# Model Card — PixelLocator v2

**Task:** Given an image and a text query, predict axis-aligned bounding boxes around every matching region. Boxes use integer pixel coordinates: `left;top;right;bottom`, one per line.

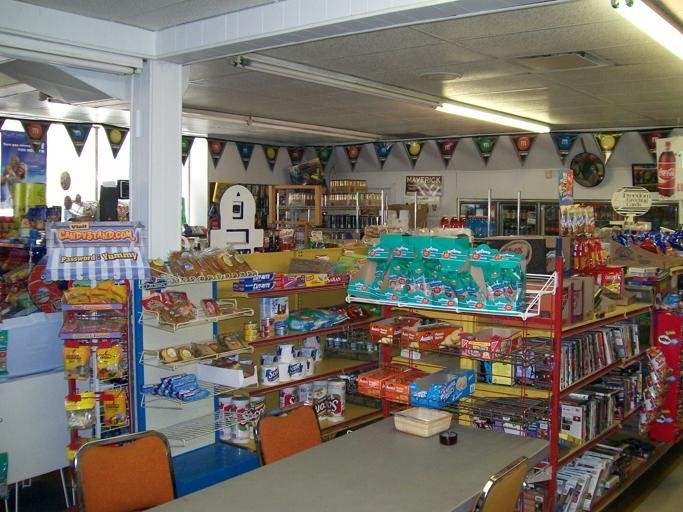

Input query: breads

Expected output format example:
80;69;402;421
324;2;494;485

159;334;245;364
149;257;167;282
171;247;255;278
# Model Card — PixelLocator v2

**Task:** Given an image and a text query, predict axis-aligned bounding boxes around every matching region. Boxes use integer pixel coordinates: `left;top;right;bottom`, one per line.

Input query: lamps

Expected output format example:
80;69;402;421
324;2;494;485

234;54;438;106
614;0;683;61
434;98;553;137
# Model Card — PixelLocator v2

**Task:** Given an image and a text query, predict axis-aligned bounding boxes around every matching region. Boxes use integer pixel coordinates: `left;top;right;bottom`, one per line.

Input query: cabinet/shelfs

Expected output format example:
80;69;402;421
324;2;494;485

0;181;683;512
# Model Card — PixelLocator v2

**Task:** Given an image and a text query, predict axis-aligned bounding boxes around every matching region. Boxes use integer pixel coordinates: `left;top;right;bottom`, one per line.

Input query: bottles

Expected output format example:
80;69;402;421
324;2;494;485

503;209;535;236
440;215;497;238
288;179;382;209
657;141;676;197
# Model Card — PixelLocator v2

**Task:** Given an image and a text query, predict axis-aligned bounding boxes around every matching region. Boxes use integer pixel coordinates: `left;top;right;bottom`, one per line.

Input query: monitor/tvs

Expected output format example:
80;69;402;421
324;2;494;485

632;162;659;192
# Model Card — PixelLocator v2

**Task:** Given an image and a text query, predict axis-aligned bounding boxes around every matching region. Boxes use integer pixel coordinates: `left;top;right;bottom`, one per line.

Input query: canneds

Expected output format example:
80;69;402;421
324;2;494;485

242;318;275;343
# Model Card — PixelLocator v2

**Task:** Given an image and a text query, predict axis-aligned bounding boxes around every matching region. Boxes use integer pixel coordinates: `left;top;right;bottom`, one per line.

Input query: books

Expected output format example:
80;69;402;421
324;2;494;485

627;266;661;286
472;315;657;512
